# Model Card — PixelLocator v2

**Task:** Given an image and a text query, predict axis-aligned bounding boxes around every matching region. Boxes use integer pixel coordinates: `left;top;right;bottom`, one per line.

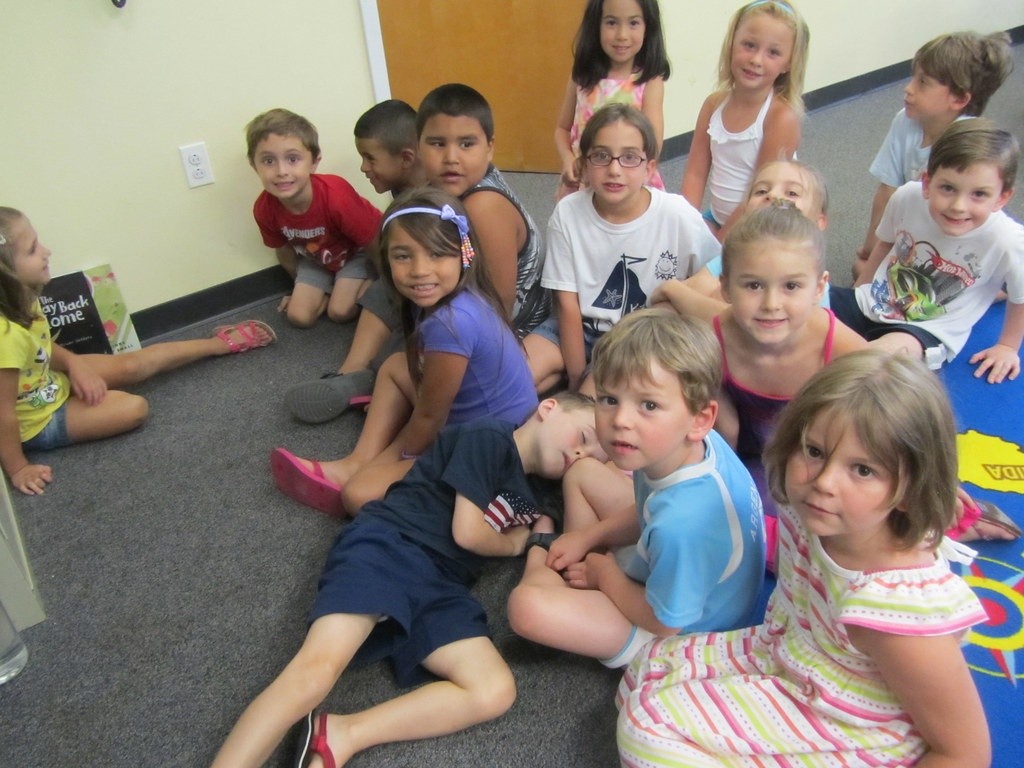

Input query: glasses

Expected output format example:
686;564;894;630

583;150;644;168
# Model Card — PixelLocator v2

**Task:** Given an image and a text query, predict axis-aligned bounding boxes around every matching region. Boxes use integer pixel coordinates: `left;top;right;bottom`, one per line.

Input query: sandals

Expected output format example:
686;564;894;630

213;319;277;353
293;708;337;768
972;500;1022;542
525;505;563;551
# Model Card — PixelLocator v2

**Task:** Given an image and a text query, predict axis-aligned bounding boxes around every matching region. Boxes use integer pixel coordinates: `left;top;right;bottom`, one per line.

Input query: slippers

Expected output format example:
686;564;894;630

272;446;346;518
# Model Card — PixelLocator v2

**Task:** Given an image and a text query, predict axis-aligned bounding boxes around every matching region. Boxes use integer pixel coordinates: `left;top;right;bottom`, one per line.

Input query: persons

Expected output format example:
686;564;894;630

211;3;1024;768
1;206;277;495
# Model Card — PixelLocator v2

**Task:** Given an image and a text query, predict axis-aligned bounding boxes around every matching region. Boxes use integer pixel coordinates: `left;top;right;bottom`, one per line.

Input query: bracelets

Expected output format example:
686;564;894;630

526;533;560;551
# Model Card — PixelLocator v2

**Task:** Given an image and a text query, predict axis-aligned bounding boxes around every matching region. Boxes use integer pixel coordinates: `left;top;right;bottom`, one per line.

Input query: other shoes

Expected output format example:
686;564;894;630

285;370;377;424
0;646;29;685
